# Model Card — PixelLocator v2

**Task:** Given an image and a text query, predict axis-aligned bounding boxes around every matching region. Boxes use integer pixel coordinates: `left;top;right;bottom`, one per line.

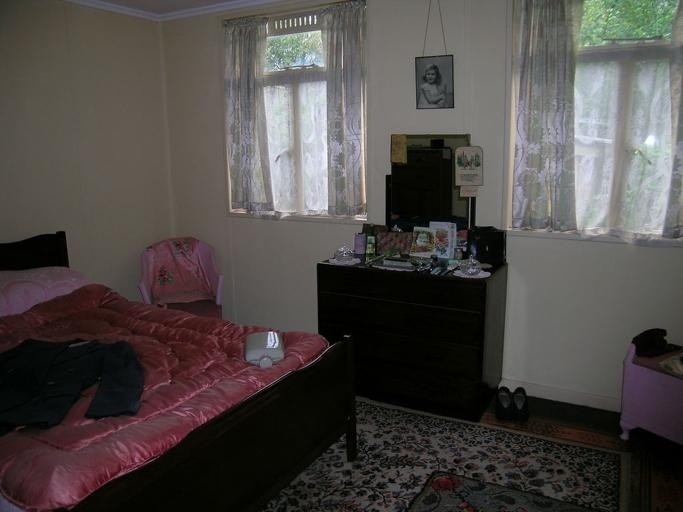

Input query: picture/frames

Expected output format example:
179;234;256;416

414;54;455;109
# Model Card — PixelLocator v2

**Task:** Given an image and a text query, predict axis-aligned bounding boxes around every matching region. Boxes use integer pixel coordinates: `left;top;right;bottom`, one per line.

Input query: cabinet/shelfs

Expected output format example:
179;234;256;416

316;260;509;422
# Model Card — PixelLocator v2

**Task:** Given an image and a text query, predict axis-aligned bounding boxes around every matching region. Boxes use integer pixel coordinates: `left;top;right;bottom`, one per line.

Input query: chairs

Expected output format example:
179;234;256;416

135;236;223;322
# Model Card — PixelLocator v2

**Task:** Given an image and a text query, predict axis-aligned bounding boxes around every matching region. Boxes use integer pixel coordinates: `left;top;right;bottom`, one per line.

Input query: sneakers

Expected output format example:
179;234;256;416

495;387;530;424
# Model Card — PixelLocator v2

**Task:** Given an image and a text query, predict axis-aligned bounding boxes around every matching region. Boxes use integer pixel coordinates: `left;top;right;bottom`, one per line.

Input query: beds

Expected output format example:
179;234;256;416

0;231;359;512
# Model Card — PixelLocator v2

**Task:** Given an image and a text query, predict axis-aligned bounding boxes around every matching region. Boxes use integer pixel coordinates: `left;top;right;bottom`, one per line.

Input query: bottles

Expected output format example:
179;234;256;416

243;331;285;369
364;224;376;256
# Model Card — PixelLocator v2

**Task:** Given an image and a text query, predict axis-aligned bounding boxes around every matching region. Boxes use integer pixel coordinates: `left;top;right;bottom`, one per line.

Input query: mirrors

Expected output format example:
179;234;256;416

390;133;472;229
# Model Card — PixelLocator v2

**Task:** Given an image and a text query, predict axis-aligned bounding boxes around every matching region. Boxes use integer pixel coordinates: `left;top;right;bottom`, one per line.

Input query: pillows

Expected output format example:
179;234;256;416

0;267;86;317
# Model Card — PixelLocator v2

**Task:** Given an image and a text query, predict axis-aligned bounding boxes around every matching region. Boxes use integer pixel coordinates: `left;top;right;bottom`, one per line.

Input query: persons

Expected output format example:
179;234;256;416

415;64;450;108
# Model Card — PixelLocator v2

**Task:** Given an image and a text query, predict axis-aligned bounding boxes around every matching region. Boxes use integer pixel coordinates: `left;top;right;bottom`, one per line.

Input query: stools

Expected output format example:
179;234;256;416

619;327;683;444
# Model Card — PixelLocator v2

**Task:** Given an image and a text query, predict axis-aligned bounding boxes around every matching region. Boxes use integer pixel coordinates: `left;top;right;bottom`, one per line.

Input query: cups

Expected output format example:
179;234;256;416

353;233;366;255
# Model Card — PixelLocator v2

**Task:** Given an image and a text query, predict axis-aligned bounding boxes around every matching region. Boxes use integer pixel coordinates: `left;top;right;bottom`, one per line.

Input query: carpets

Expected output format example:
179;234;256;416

261;396;631;512
